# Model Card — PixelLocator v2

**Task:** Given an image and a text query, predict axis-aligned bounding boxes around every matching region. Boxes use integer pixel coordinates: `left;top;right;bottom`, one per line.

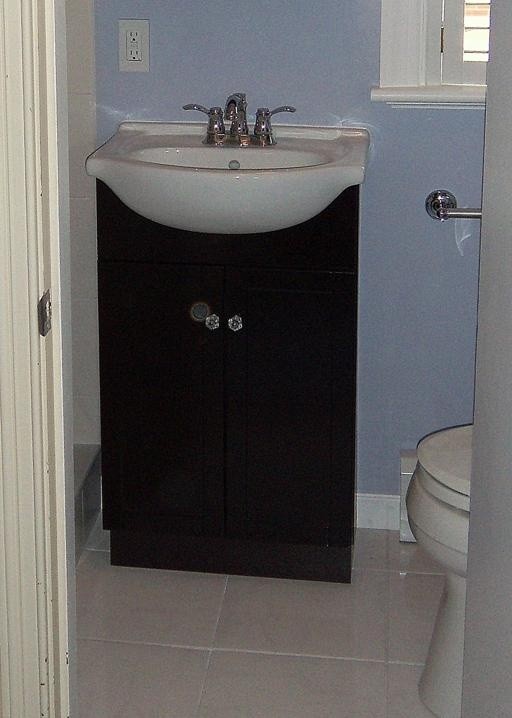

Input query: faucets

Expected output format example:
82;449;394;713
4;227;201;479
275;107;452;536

223;91;250;139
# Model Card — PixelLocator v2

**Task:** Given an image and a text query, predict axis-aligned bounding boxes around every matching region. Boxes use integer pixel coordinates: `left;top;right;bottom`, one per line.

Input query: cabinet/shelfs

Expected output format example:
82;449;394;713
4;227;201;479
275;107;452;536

96;177;361;583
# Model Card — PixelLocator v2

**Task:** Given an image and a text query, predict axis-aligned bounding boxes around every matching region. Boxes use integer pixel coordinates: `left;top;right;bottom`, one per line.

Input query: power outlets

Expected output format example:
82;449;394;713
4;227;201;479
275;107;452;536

116;19;152;73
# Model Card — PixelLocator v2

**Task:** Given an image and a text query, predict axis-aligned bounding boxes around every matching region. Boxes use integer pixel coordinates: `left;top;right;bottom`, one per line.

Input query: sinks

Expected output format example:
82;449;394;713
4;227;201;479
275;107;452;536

85;122;369;235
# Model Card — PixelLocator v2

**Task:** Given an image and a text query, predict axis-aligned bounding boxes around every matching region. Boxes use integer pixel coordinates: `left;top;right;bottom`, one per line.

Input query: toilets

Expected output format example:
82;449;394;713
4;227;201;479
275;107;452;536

406;420;473;718
